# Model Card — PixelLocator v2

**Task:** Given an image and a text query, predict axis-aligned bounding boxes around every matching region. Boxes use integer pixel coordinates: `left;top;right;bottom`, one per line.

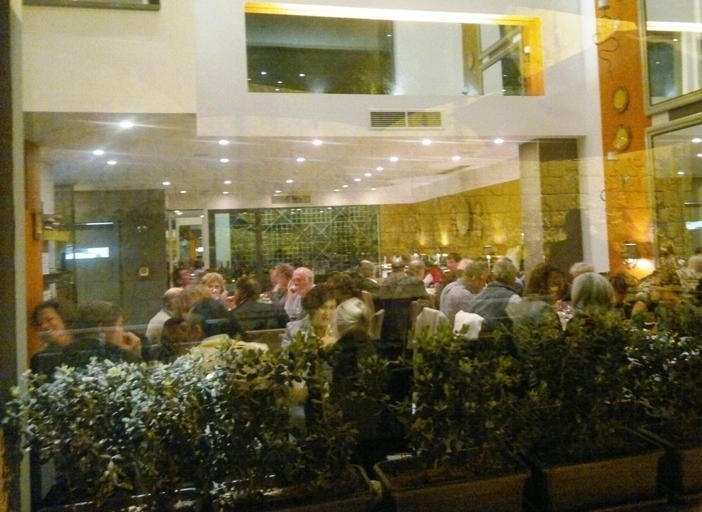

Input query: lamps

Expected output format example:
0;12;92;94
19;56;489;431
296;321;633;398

596;1;621;72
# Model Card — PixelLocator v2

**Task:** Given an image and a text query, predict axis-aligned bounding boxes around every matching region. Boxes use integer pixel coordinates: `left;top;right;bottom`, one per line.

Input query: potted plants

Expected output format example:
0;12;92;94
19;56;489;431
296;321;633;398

632;322;701;498
527;313;663;512
374;324;526;511
1;330;374;512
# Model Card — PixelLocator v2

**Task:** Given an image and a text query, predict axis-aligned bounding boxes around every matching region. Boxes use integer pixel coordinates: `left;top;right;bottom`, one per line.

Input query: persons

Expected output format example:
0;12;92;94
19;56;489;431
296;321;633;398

30;237;700;474
